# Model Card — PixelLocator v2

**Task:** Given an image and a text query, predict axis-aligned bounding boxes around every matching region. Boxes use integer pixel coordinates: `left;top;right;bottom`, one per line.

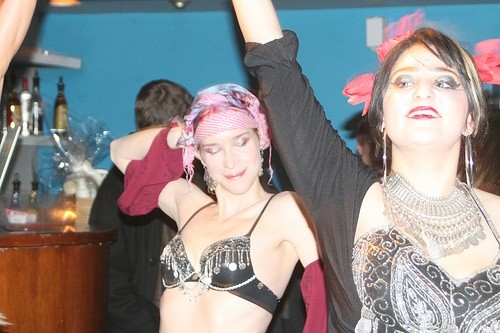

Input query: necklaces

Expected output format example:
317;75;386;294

381;168;487;260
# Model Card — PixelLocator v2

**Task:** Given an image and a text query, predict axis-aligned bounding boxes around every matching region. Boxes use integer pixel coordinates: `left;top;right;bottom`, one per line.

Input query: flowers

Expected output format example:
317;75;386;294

473;39;500;85
376;9;424;64
341;73;376;116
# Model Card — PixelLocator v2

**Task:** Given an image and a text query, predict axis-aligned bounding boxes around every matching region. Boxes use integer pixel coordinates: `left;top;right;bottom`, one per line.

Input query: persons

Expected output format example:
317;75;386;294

0;0;37;102
89;80;327;333
233;0;500;333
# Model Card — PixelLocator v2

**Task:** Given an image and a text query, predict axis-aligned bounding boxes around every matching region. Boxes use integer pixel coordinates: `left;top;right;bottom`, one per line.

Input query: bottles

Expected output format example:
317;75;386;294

54;77;70;130
10;172;24;231
28;182;39;231
5;68;42;135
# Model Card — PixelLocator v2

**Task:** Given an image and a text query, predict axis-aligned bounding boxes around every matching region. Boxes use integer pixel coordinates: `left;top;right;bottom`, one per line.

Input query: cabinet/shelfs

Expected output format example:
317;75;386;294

0;51;82;147
